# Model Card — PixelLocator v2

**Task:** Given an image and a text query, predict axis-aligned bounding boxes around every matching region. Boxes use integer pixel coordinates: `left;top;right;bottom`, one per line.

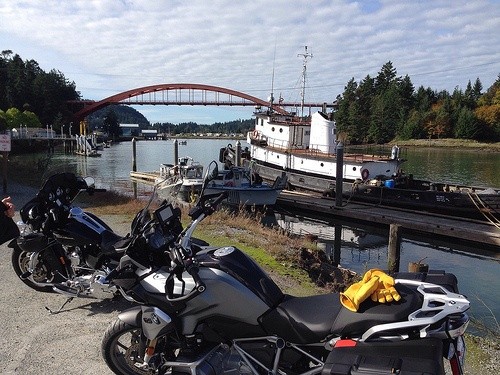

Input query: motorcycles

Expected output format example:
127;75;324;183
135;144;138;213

7;171;210;315
101;160;471;375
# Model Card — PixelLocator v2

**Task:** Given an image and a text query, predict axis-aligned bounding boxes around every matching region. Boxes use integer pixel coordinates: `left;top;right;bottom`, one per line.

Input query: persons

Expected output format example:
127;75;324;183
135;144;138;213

0;197;21;246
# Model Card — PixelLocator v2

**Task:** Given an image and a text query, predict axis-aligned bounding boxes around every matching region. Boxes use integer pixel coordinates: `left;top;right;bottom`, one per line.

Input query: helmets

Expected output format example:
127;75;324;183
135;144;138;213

16;233;48;252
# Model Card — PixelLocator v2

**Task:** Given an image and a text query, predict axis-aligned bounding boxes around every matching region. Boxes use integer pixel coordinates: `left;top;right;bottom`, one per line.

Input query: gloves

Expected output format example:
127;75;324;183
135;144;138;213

340;268;401;312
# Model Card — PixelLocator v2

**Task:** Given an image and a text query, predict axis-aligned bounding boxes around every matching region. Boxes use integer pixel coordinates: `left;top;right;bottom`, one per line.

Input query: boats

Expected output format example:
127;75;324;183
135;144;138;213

202;166;288;214
152;157;204;208
219;46;500;225
